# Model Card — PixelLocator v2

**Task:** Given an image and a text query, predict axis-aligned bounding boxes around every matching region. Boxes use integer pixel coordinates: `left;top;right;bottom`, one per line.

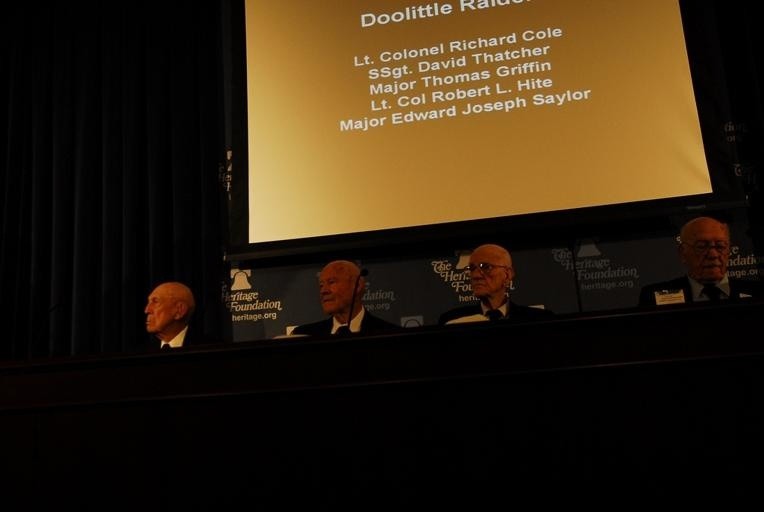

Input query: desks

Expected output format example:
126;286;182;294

0;295;764;512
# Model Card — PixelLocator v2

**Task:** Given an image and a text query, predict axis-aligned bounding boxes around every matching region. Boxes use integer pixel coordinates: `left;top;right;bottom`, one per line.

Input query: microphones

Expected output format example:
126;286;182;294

29;303;61;357
335;269;368;337
567;242;583;313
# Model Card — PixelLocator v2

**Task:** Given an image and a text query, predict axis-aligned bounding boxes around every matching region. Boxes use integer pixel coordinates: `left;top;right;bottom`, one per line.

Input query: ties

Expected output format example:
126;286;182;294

700;285;722;301
484;309;503;321
160;344;171;351
333;325;351;336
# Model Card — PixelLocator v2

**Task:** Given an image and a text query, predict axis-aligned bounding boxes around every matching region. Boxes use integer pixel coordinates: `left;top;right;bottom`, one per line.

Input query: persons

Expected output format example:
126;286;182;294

122;281;223;355
439;244;556;328
290;259;404;343
639;216;761;314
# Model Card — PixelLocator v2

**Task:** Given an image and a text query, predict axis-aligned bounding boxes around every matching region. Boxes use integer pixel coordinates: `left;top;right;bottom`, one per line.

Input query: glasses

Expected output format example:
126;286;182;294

683;239;731;253
462;261;508;276
